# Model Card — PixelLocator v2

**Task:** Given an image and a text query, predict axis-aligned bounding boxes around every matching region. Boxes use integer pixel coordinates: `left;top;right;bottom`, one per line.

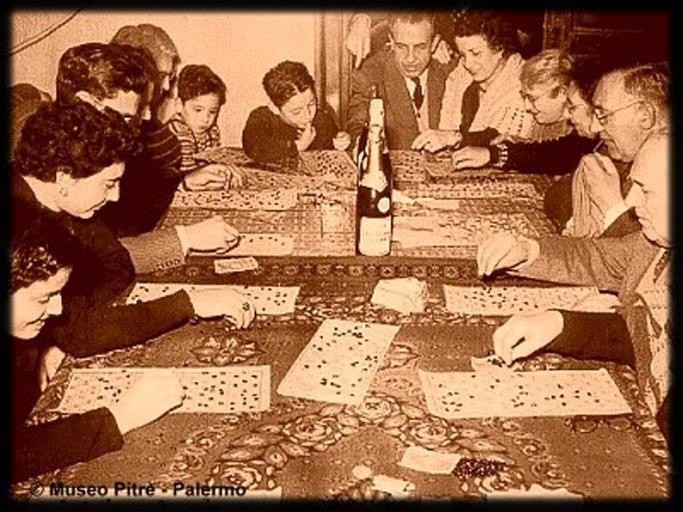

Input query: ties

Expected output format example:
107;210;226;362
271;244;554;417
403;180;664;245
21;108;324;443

411;77;424;107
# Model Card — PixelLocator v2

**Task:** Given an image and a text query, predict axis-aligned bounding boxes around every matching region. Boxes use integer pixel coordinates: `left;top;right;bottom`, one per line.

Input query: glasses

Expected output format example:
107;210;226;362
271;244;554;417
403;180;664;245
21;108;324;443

593;101;643;124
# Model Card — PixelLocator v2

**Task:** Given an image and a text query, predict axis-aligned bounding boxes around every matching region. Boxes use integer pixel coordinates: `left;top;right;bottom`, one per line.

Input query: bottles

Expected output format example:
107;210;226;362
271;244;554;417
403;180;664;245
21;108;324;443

355;83;393;258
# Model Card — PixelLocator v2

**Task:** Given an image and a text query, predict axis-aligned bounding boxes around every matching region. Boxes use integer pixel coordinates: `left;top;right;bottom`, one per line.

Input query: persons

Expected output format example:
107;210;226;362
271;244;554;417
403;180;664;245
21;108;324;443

10;12;672;484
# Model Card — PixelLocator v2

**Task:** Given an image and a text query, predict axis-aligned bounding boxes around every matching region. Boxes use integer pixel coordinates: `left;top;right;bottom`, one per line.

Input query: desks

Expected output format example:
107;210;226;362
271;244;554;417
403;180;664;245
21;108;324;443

15;147;674;496
